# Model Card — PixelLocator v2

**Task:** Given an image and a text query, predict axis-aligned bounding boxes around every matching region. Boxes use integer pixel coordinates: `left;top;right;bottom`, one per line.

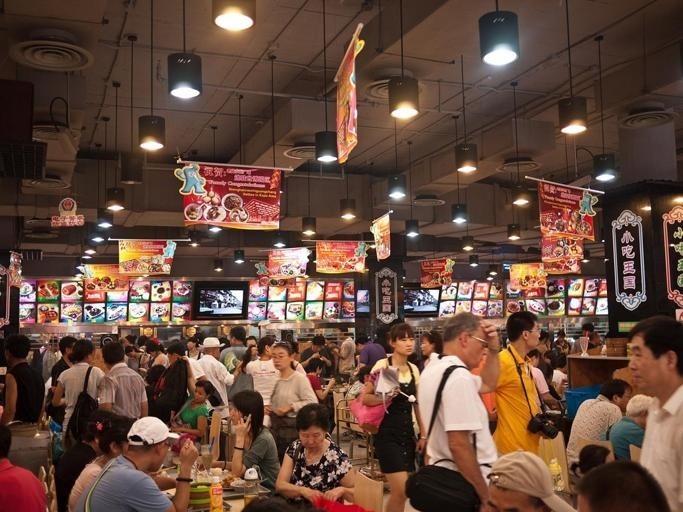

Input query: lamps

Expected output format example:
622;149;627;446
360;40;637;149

452;55;477;226
559;2;620;183
74;80;126;280
208;1;257;33
506;84;530;242
190;224;245;271
271;159;357;249
388;1;419;239
123;2;202;185
478;2;519;66
315;1;337;163
461;235;510;280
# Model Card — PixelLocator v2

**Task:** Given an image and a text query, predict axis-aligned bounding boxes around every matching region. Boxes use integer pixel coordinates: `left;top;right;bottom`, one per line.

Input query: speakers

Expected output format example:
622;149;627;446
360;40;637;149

281;330;293;342
101;334;118;347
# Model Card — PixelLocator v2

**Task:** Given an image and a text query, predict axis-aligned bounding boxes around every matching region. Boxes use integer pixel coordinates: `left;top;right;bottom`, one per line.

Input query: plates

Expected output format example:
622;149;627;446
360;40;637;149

322;382;350;393
552;207;591;271
318;258;360;270
183;191;250;222
439;284;607;314
20;283;190;320
223;487;230;491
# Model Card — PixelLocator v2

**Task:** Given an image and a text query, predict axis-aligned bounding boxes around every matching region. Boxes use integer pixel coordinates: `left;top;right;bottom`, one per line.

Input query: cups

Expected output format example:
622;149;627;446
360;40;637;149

250;286;340;318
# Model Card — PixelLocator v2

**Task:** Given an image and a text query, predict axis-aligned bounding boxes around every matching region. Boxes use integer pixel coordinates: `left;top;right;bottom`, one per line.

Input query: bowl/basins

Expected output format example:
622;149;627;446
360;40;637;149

231;480;244;492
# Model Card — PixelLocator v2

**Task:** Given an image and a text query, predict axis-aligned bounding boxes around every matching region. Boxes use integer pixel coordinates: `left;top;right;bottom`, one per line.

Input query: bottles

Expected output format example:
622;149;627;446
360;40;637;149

548;457;565;492
243;468;260;505
210;476;224;512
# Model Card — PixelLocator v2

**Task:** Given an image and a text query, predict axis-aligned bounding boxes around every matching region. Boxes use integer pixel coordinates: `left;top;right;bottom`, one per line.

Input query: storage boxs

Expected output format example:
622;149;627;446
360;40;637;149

563;384;617;421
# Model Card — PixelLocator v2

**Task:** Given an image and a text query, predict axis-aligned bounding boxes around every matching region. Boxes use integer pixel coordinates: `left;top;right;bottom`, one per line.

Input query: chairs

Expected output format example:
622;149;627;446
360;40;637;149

39;460;63;512
336;398;369;466
200;411;235;461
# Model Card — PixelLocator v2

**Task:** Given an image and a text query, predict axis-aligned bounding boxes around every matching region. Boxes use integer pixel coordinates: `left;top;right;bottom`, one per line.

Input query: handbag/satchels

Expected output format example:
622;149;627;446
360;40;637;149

63;365;99;450
403;458;482;512
44;387;66;418
229;373;254;401
277;426;300;450
348;383;393;435
168;422;197;456
5;419;43;439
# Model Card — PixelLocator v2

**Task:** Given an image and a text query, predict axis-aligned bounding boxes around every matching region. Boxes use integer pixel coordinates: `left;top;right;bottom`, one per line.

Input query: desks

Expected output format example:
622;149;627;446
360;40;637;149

162;451;271;512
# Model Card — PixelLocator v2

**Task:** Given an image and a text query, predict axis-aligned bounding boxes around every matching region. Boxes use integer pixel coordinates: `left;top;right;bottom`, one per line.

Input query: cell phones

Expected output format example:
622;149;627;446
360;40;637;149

243;416;251;424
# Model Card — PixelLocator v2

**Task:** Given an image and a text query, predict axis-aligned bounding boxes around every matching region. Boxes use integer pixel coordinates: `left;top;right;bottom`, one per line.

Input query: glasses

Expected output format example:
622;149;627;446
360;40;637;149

471;336;489;347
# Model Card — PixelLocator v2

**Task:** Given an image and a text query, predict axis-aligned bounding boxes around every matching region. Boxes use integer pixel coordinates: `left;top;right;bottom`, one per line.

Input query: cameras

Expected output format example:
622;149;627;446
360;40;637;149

527;413;560;439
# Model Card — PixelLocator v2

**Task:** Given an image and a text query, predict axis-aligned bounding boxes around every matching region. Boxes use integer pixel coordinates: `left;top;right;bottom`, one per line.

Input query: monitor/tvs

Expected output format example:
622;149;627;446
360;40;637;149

403;287;441;317
192;281;249;319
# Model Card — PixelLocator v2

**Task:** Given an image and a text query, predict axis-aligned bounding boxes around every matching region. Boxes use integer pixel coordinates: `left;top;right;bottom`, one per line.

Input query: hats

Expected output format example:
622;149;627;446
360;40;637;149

198;336;227;349
488;450;579;512
125;416;182;447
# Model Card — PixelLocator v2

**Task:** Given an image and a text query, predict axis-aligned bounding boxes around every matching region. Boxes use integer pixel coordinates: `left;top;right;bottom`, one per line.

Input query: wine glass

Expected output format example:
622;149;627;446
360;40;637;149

201;444;213;482
578;336;593;357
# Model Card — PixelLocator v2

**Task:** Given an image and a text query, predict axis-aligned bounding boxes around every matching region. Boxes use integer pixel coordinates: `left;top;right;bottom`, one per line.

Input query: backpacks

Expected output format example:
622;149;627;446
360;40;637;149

151;359;188;412
